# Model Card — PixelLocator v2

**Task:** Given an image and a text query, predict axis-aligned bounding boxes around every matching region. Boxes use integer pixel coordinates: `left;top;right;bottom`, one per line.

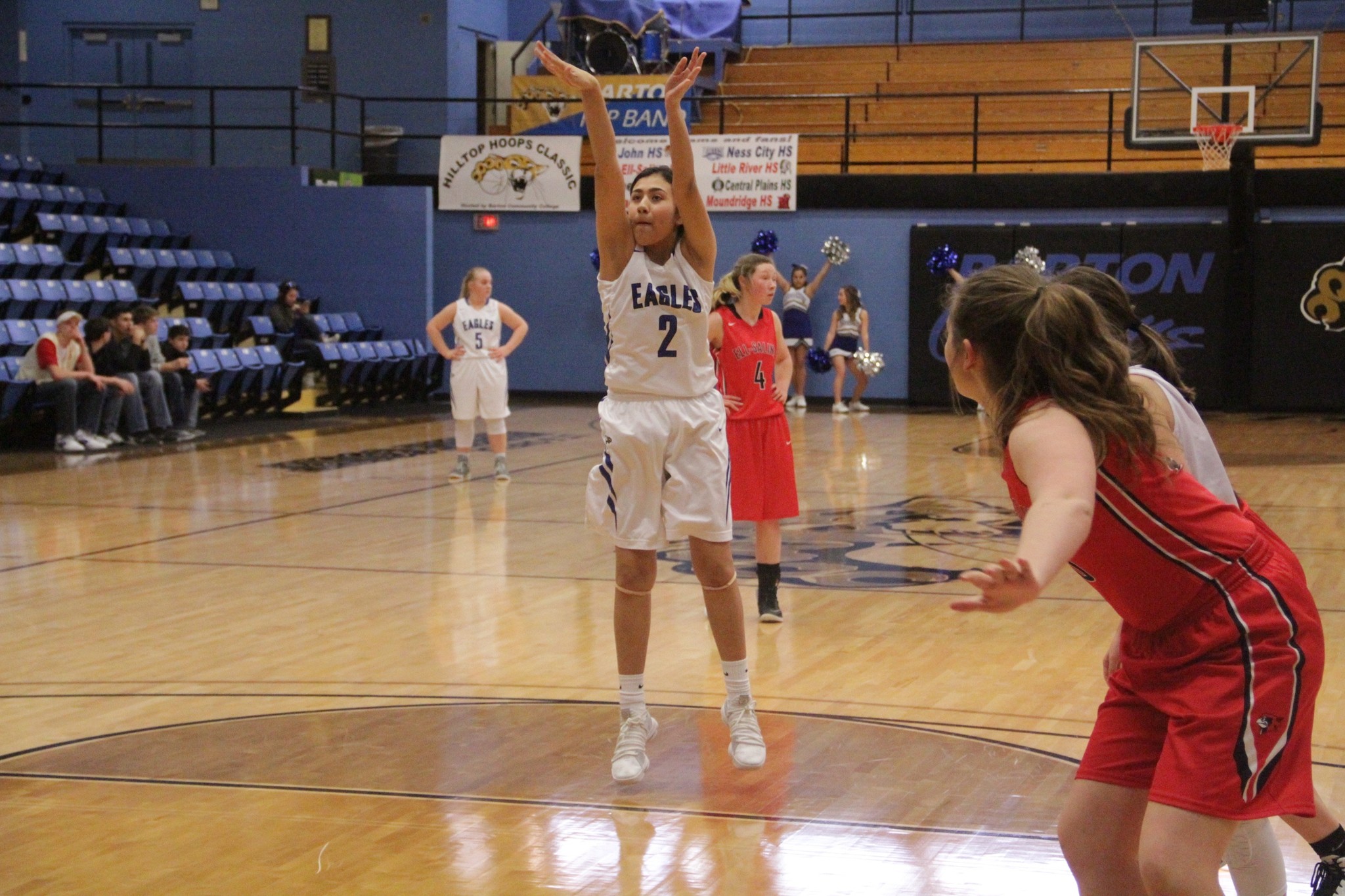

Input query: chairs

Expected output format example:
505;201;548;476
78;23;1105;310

0;155;435;457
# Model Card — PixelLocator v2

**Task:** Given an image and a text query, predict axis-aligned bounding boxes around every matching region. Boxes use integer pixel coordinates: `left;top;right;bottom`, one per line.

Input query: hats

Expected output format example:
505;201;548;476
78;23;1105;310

56;311;83;327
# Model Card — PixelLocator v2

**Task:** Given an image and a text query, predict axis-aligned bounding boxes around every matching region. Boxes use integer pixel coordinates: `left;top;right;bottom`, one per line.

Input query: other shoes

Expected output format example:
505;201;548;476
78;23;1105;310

53;429;207;452
304;372;316;389
323;333;340;344
832;403;849;413
849;400;870;411
796;396;806;408
786;395;797;407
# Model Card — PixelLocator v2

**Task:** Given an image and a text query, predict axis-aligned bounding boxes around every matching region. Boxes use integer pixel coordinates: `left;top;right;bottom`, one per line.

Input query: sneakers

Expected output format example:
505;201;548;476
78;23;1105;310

757;587;784;623
448;455;472;479
494;456;510;480
611;708;659;785
720;695;766;770
1310;855;1345;896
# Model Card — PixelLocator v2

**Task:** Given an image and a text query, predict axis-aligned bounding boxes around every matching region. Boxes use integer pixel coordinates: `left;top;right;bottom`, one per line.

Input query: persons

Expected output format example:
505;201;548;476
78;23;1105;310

1048;265;1344;895
763;253;834;406
14;299;213;453
941;260;1326;896
426;265;529;480
821;285;871;412
271;279;340;344
532;39;766;782
706;253;799;624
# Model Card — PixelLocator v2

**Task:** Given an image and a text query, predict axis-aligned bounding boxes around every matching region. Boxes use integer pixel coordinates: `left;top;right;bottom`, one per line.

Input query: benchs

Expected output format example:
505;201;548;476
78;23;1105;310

691;31;1345;177
